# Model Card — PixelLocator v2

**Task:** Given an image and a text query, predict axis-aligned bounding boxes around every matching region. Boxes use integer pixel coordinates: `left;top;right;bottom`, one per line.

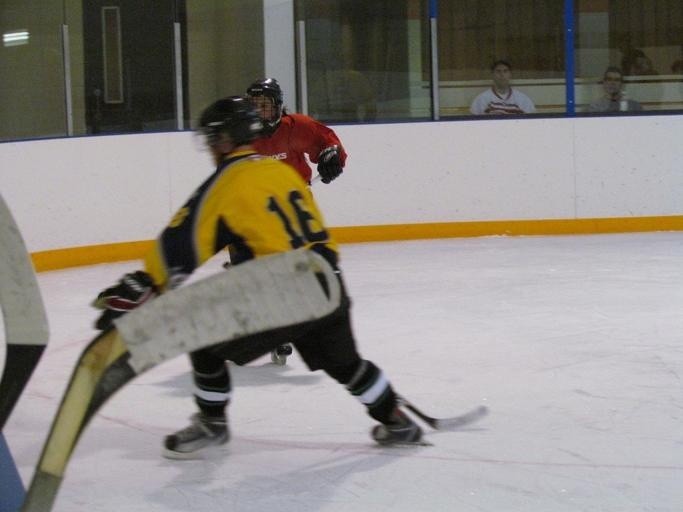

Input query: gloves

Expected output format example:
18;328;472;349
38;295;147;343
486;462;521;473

317;145;343;184
92;272;157;328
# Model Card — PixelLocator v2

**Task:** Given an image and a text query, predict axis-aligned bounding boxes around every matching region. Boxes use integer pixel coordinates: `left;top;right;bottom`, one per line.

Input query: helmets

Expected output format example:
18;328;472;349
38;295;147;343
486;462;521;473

195;97;262;151
244;77;282;132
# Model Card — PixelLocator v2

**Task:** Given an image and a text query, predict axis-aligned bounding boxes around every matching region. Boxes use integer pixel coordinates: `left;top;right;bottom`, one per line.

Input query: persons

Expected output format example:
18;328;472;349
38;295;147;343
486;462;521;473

246;77;347;354
584;66;643;114
90;95;422;456
469;59;537;115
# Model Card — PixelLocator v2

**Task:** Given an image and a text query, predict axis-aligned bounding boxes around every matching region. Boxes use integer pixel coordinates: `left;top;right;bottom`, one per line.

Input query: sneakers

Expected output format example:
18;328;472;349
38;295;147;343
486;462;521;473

372;422;421;446
165;416;228;453
276;341;292;357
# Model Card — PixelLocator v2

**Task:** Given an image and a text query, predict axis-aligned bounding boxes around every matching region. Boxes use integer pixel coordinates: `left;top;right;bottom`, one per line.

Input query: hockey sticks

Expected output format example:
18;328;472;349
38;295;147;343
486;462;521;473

391;391;490;432
20;247;342;510
0;195;52;430
